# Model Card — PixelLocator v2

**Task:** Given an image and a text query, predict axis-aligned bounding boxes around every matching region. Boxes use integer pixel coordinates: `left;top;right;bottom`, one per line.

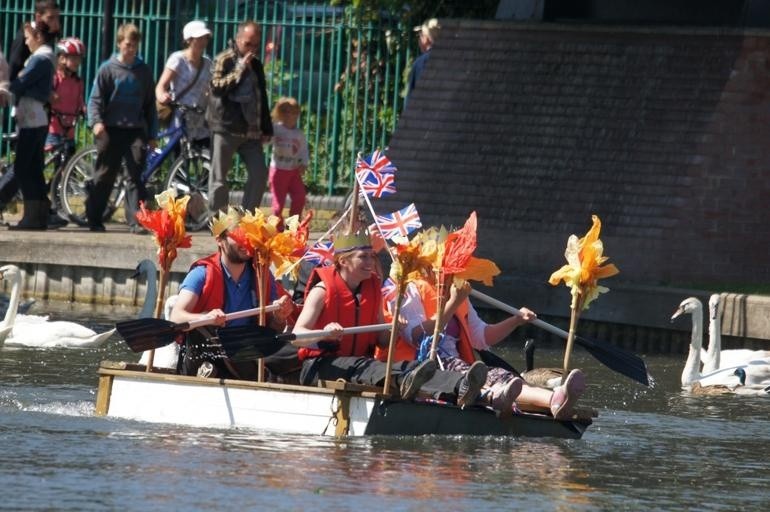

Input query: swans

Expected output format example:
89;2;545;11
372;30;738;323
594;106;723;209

0;264;118;349
701;293;770;375
670;296;769;389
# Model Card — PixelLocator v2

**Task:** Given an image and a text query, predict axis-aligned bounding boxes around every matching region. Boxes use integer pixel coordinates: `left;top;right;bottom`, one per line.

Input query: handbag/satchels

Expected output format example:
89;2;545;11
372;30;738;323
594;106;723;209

155;58;206;124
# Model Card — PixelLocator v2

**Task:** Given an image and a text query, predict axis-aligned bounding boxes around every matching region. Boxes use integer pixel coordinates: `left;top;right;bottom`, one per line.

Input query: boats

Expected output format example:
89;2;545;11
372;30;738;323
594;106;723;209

94;357;596;448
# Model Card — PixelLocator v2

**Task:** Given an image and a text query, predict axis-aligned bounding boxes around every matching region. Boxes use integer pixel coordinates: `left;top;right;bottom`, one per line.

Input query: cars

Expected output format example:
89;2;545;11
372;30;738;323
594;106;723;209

262;28;401;116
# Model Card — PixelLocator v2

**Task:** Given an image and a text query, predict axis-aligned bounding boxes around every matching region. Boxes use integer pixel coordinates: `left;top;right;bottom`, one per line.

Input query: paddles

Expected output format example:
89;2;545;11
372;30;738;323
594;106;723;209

216;323;392;361
116;304;280;352
470;288;649;386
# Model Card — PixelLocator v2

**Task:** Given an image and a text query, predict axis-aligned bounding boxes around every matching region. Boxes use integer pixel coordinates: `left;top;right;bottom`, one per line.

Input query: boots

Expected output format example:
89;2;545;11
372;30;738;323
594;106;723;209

9;198;53;230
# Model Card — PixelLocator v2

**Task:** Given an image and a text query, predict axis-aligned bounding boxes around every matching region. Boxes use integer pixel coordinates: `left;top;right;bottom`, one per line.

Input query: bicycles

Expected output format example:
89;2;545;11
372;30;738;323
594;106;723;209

2;104;100;223
61;98;216;231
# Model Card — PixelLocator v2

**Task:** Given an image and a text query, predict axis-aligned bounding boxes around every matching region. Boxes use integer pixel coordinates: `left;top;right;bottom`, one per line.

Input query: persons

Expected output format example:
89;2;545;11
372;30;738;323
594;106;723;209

1;3;440;232
164;209;587;420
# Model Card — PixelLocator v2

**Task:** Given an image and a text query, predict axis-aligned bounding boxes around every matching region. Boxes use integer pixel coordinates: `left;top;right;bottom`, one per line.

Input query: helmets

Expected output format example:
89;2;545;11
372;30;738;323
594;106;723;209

55;36;86;57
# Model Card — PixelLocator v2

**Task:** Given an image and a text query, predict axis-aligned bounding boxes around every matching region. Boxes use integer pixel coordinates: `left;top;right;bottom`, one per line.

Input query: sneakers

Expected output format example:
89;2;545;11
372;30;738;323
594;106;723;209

456;359;486;410
491;376;522;420
130;222;150;234
88;217;105;232
399;357;437;401
549;367;586;422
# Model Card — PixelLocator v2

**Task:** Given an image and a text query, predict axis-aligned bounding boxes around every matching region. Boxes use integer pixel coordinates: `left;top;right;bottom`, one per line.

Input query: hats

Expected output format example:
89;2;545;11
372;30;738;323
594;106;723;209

181;20;212;43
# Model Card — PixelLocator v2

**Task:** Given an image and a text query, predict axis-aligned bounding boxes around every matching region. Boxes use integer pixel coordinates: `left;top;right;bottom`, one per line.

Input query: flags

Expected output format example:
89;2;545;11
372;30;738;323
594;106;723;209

302;152;423;286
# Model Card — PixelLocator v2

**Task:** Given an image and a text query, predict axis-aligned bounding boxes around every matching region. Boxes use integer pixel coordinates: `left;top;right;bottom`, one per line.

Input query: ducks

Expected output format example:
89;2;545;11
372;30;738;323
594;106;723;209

727;367;770;395
